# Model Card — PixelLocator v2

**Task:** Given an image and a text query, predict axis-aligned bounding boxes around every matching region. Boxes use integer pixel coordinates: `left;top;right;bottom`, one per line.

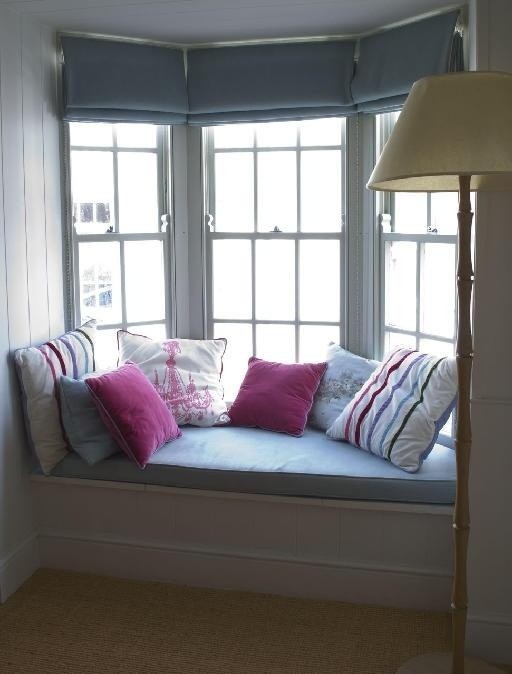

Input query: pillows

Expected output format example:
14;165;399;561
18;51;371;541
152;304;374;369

117;330;231;428
307;342;380;430
227;355;327;439
324;343;460;473
12;318;97;477
58;365;122;467
85;359;182;470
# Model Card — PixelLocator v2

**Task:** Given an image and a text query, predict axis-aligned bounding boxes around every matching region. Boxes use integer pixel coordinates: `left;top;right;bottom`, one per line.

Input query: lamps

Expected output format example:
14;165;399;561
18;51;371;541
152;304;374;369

365;70;512;674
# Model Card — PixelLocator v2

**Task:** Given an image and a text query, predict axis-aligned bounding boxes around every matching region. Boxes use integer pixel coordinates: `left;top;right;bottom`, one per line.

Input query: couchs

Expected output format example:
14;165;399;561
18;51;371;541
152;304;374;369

24;427;459;616
